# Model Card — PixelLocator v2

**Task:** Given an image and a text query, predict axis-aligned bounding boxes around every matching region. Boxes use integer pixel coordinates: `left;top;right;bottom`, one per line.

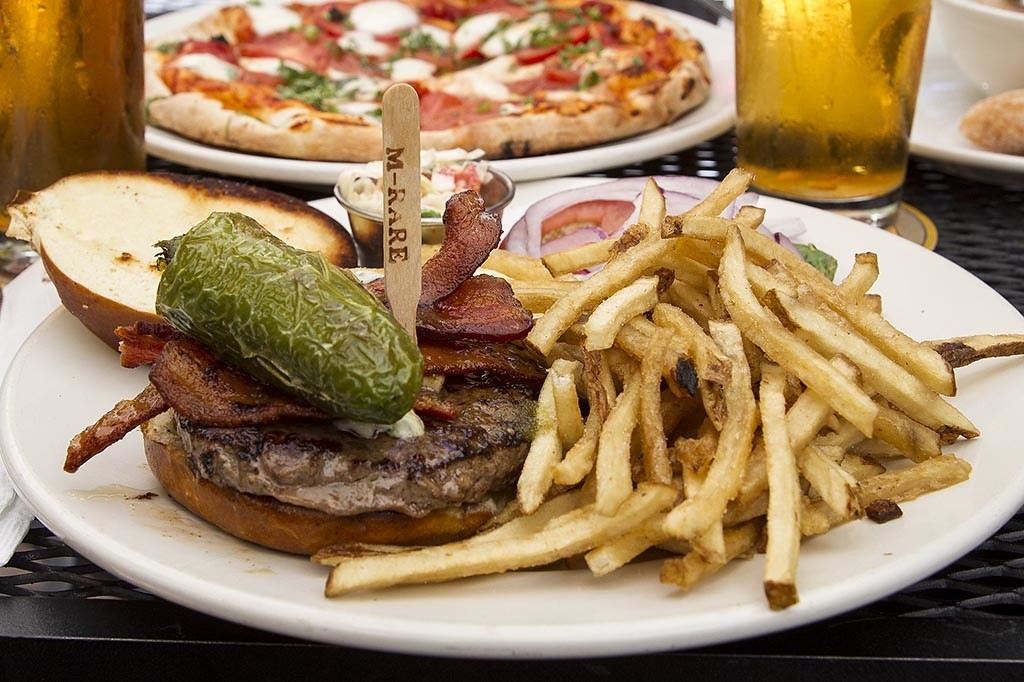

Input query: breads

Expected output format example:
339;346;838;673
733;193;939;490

959;84;1024;158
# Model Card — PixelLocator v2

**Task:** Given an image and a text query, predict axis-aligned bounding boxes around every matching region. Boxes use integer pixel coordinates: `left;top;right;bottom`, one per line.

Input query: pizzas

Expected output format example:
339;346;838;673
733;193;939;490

144;0;711;161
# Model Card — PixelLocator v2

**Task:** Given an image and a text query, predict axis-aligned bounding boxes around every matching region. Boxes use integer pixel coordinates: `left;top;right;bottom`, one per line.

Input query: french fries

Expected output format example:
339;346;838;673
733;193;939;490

310;168;1023;610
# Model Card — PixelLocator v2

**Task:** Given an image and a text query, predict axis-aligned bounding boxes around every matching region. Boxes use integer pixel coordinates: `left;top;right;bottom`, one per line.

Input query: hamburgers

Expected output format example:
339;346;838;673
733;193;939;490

7;170;560;558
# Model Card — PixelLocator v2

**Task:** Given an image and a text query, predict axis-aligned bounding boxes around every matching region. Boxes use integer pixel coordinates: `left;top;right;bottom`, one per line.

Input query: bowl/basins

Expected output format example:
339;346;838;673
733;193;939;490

936;0;1024;114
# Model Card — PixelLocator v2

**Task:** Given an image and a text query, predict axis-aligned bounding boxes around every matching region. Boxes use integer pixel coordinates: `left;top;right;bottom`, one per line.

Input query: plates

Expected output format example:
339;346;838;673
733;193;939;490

906;47;1024;188
0;193;1024;660
140;0;738;187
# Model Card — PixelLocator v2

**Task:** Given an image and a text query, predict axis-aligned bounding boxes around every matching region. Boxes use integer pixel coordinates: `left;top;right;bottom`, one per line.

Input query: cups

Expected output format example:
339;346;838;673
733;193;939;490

732;0;934;229
0;0;146;227
